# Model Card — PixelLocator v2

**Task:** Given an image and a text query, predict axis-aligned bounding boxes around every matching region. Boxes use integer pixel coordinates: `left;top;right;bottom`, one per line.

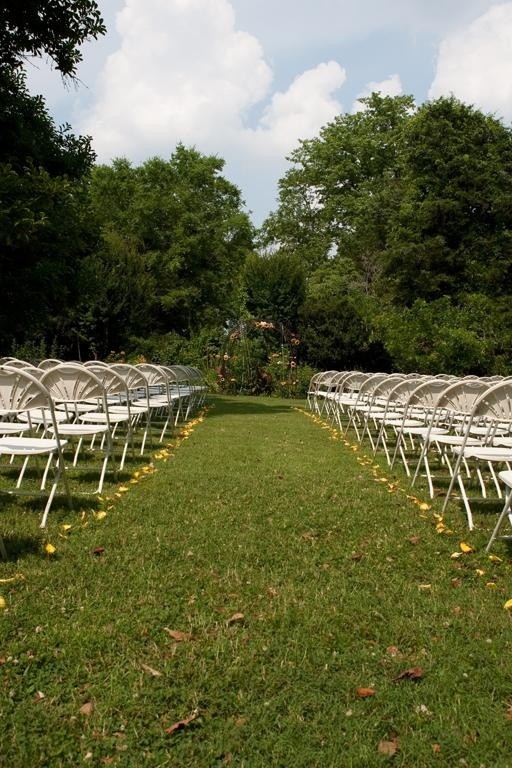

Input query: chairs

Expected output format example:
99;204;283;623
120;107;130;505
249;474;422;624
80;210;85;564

305;369;512;560
0;356;209;534
